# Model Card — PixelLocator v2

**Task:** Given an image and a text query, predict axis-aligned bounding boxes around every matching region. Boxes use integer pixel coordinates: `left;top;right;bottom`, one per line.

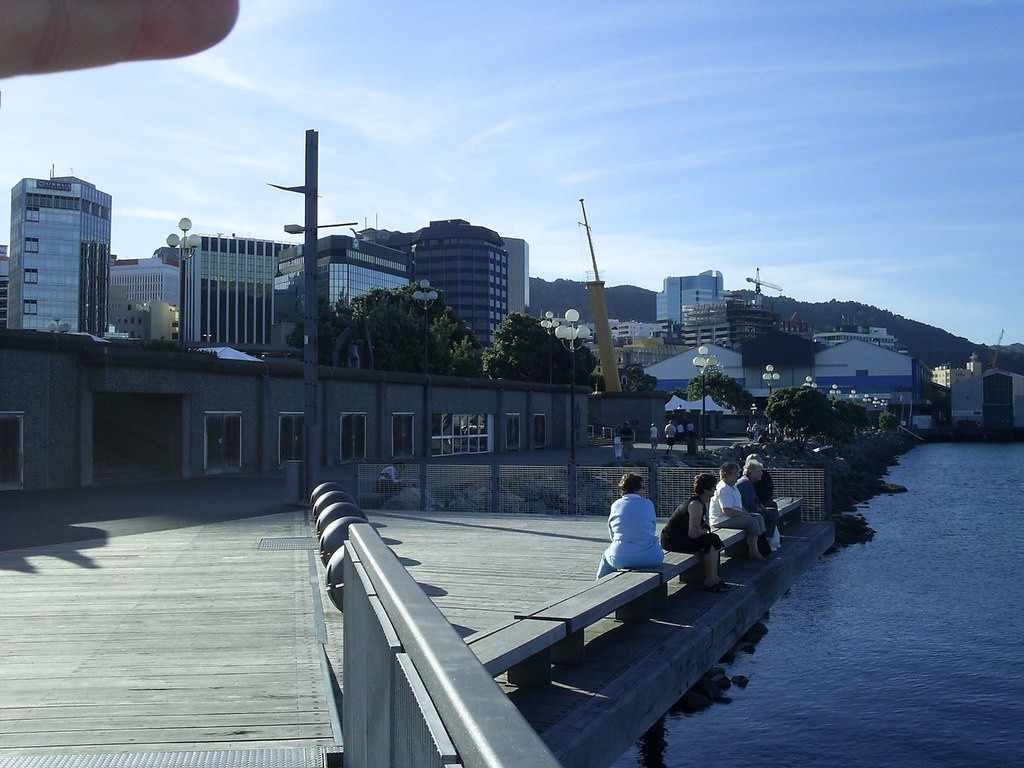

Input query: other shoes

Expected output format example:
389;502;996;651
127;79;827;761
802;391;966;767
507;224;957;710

746;529;782;564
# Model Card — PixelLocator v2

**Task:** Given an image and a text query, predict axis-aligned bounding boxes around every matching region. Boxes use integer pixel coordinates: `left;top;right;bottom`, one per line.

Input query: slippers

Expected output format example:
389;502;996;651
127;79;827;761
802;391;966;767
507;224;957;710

718;580;731;590
705;583;728;593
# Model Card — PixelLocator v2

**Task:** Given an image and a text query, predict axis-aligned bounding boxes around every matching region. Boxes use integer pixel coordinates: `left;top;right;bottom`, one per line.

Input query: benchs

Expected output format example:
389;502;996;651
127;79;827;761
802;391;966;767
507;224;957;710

463;495;803;688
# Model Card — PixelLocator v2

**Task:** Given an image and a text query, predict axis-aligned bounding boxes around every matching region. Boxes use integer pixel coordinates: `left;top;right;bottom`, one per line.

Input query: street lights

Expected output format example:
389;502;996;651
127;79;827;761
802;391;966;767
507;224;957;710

762;365;780;435
413;279;440;372
693;346;718;451
554;308;592;465
166;218;203;352
285;222;358;499
540;312;559;383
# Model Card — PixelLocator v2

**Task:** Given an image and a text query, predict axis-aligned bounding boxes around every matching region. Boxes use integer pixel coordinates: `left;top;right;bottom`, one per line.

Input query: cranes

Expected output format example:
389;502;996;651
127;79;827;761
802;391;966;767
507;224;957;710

746;267;783;306
988;329;1006;371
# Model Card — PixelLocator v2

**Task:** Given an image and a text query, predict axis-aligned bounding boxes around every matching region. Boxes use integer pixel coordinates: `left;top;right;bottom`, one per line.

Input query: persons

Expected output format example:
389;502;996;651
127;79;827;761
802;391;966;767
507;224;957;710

660;473;730;593
375;462;406;493
734;459;775;556
746;420;790;443
615;424;623;461
746;453;783;549
597;472;664;580
687;420;695;440
665;419;676;454
649;423;659;454
709;461;767;563
677;422;685;442
621;419;635;462
